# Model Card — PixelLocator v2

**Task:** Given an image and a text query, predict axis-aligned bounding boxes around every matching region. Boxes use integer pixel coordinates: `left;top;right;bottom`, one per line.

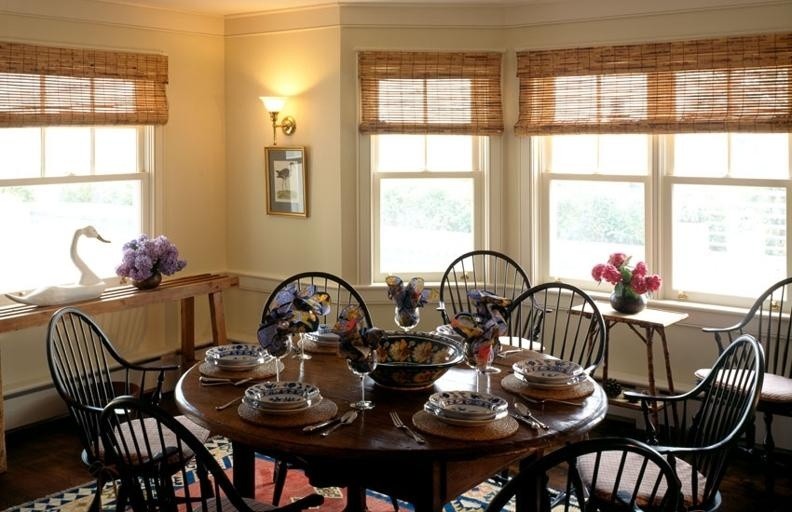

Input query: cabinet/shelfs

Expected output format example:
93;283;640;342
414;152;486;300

563;295;690;438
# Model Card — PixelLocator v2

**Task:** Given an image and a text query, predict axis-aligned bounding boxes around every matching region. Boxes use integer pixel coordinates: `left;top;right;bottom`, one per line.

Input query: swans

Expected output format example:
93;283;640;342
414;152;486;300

3;226;111;307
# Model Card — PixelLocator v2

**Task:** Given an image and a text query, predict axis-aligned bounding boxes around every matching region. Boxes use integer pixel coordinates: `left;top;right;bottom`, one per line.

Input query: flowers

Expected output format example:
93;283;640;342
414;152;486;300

118;234;186;284
589;248;663;292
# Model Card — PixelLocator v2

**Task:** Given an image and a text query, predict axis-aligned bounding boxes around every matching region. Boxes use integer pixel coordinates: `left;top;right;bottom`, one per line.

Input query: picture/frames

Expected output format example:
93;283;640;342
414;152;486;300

261;145;311;221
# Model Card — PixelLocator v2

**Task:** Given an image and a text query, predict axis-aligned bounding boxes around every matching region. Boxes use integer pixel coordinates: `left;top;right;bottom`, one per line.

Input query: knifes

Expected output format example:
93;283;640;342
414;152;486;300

303;415;342;432
509;410;539;427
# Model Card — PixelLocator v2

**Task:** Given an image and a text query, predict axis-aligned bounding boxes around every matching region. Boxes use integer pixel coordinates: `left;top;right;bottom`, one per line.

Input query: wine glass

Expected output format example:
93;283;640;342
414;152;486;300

296;331;312;360
268;333;291;382
346;347;377;410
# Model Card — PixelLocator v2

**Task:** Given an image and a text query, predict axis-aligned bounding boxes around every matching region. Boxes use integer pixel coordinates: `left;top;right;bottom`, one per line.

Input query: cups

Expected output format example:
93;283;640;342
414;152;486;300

395;306;419;331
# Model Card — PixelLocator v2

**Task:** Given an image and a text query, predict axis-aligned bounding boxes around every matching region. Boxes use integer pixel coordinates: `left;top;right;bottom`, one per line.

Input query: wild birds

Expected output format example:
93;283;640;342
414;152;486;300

276;169;290;191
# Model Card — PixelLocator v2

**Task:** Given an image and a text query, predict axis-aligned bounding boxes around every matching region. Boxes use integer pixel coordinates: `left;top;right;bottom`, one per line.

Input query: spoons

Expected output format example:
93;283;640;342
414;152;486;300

320;410;358;436
515;402;550;430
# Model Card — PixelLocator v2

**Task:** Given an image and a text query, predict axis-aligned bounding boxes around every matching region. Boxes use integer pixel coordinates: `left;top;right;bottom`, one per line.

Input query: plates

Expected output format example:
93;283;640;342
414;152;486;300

510;358;589;392
437;324;464;341
305;331;341;348
204;345;265;371
242;379;323;415
424;392;511;427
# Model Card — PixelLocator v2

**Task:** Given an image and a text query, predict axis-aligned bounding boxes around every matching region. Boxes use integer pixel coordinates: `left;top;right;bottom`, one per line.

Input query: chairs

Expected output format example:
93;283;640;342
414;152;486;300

683;277;791;452
486;436;686;510
255;272;380;504
435;251;541;340
497;284;608;374
101;388;327;512
44;304;215;511
563;333;768;509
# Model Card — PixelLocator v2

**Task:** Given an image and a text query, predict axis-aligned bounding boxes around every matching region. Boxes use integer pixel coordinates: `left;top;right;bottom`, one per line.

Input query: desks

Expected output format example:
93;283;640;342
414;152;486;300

2;270;246;477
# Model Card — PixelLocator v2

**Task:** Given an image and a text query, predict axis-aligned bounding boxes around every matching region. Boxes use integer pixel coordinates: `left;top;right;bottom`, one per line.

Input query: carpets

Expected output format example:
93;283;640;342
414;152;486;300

1;433;640;512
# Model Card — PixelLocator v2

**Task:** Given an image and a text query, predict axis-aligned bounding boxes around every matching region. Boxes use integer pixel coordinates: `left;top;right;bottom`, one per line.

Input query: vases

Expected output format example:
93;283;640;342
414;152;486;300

135;269;167;290
610;289;651;314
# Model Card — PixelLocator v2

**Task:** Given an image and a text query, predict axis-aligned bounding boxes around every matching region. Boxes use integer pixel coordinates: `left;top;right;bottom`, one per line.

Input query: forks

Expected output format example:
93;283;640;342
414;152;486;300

498;348;522;358
203;376;257;386
389;409;425;445
518;392;582;408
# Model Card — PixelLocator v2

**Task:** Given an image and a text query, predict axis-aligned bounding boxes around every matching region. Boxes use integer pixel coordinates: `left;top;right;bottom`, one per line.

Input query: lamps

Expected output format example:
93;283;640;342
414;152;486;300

257;93;304;145
